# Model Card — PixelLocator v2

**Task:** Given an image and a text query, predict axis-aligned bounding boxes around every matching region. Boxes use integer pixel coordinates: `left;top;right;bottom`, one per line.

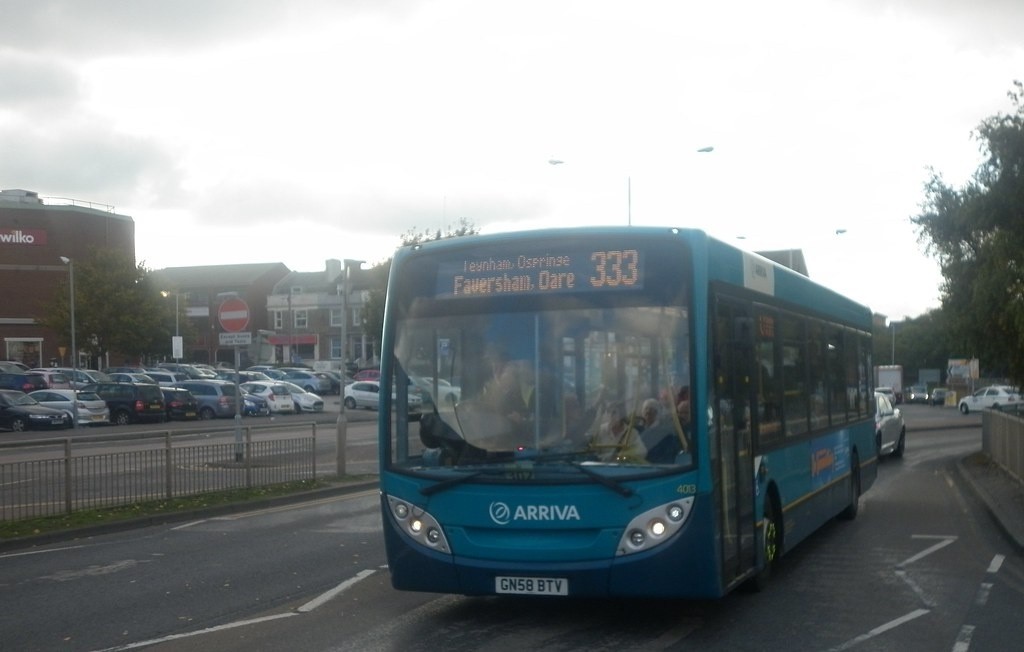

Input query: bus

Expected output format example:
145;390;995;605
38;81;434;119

378;226;877;599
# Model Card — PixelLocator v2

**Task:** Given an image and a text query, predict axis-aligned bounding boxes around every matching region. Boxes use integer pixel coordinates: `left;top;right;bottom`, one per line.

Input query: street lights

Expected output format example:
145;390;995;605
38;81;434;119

735;228;848;269
547;145;715;228
337;259;367;477
287;291;303;366
60;256;79;429
161;290;178;374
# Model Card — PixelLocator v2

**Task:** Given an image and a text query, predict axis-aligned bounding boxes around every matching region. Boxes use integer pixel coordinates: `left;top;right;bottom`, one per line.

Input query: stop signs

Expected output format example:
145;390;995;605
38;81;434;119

218;299;248;332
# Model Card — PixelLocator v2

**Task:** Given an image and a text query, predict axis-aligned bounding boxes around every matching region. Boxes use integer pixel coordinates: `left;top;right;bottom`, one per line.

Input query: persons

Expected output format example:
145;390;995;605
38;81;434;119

464;360;535;425
565;384;605;448
600;401;648;462
811;394;824;418
677;386;690;422
642;398;661;427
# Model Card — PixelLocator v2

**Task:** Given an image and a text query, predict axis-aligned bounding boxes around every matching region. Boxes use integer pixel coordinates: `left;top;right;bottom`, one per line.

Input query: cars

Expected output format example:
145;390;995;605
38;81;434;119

0;389;68;432
392;376;432;403
171;380;245;420
276;370;331;395
873;392;906;458
26;370;71;390
0;373;50;394
0;360;31;372
344;381;423;411
106;373;156;383
423;377;462;405
282;381;324;413
314;371;353;394
146;362;313;387
102;367;145;373
240;387;271;416
80;381;167;426
240;381;294;413
929;389;948;405
83;370;111;382
907;386;929;403
874;387;896;406
35;367;98;390
160;387;200;421
352;370;379;381
958;386;1024;415
17;389;110;428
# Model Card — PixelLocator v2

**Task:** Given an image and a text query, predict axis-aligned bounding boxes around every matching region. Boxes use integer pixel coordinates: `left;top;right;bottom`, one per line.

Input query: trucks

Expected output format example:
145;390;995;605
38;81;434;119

874;365;903;404
